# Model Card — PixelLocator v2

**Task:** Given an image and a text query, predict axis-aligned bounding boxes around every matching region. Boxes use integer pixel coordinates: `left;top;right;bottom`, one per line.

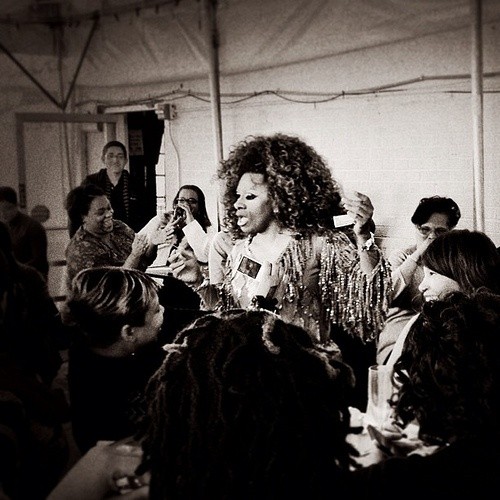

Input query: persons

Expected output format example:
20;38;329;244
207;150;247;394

376;195;500;500
0;140;356;500
209;132;394;362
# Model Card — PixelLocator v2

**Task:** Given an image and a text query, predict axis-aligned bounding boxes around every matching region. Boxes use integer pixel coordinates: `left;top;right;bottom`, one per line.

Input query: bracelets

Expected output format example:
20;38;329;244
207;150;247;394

356;231;375;251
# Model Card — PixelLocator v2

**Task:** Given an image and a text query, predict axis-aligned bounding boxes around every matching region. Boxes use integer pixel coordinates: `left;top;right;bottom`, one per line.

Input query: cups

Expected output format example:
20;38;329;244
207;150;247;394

367;365;397;433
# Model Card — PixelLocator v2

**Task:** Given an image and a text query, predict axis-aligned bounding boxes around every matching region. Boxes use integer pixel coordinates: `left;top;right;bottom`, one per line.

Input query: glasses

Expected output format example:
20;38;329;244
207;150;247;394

175;197;199;206
417;224;448;238
